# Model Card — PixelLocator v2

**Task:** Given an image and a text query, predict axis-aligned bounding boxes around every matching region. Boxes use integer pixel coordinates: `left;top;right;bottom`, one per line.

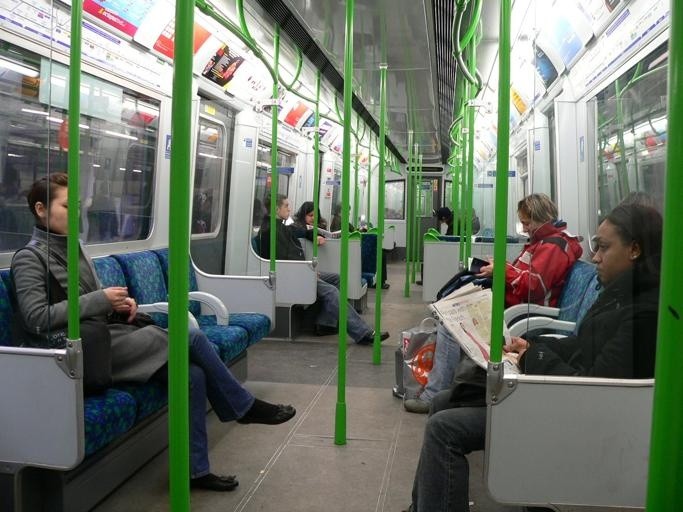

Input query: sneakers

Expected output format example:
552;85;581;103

415;277;424;286
356;330;390;346
370;281;391;291
405;396;451;413
311;325;340;337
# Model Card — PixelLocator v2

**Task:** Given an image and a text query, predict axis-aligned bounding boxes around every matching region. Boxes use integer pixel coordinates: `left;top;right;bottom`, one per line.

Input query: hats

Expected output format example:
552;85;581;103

431;206;452;220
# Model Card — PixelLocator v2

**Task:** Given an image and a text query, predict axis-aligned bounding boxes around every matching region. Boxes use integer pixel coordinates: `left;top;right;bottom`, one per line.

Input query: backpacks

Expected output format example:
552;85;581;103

447;215;481;235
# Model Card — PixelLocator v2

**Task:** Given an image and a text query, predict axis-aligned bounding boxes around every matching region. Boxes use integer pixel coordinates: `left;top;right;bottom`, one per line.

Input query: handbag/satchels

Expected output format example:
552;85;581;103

393;314;442;400
9;247;112;397
432;269;492;302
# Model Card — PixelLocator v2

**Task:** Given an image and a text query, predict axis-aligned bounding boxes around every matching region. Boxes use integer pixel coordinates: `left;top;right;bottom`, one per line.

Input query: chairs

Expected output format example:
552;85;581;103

475;227;608;511
0;245;271;511
297;232;376;300
252;234;316;308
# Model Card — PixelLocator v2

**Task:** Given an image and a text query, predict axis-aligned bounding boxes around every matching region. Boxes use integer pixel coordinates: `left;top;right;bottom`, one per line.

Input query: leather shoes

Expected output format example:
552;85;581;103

190;470;239;492
236;399;298;426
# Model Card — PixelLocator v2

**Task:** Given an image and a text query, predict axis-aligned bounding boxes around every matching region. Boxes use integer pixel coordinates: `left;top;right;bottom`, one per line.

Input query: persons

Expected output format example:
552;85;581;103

254;199;265;226
293;201;326;232
405;193;584;413
330;203;390;289
472;208;480;235
405;206;662;511
415;207;461;285
256;193;390;345
0;165;28;205
86;182;118;245
9;173;296;492
201;197;213;232
